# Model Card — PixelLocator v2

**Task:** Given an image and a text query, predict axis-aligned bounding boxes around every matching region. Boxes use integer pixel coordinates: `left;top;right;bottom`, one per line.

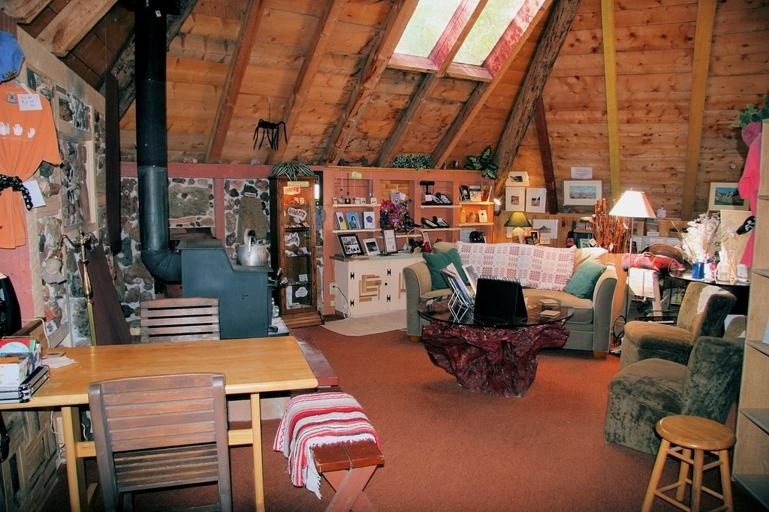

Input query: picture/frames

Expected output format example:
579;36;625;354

707;180;748;212
338;228;399;256
500;170;559;245
562;177;602;207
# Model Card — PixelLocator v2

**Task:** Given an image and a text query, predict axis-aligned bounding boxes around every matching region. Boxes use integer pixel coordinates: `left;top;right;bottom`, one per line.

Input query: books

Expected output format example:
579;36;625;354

539;309;561;319
440;262;473;305
539;299;558;307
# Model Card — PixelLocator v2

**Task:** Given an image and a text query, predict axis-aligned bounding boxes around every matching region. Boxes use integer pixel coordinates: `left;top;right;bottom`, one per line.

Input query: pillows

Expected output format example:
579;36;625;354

563;259;606;300
421;247;466;292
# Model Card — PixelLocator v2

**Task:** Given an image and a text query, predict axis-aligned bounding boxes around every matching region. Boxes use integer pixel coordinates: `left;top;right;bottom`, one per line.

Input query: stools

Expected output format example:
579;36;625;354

641;413;740;512
293;335;340;389
294;386;384;512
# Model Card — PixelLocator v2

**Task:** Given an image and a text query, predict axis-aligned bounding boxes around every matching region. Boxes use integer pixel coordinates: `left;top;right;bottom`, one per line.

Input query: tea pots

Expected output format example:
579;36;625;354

237;230;267;267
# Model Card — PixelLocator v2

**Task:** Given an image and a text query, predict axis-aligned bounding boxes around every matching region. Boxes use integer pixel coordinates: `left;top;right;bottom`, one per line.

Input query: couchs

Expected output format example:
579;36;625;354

403;241;619;361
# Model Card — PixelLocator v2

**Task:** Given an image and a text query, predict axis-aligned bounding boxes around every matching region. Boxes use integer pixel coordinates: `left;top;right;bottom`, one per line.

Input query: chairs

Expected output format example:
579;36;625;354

84;370;234;512
140;298;220;341
602;313;748;459
619;280;737;370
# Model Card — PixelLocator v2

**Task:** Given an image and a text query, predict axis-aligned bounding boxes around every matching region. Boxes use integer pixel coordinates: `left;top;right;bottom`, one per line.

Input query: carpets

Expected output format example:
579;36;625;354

320;309;409;337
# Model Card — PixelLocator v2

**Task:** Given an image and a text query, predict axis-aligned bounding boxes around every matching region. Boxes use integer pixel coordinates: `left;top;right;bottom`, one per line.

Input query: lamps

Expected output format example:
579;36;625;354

608;187;657;256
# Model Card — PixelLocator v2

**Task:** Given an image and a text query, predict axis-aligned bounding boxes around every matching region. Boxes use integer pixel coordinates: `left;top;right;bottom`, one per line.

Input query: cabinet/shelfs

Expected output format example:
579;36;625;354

270;175;317;315
329;168;493;244
729;120;768;512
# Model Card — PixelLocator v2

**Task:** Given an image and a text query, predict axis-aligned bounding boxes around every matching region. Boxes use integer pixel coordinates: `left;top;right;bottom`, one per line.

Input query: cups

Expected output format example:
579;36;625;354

692;262;704;279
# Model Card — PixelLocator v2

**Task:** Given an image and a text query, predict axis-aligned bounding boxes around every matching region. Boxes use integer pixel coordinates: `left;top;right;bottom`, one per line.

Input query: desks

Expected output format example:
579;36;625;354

330;252;425;320
1;336;319;512
668;268;749;316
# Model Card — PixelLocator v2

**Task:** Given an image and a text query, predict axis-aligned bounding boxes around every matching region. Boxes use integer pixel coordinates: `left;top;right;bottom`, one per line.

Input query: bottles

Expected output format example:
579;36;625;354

460;204;466;224
469;211;476;223
345;191;351;204
